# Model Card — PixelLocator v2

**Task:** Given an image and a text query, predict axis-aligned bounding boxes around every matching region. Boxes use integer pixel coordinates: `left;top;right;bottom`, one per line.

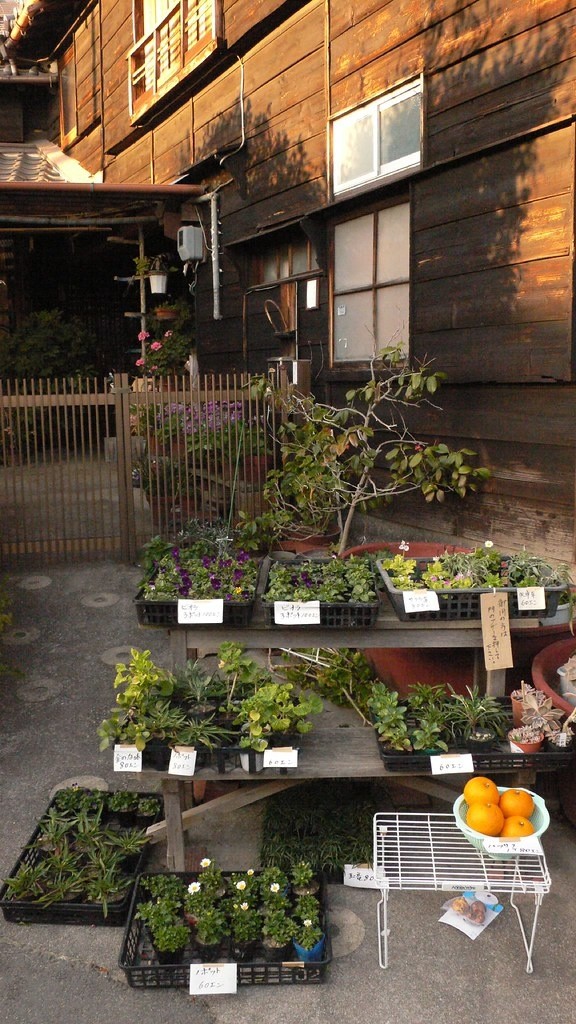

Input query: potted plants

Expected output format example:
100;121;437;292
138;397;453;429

368;680;509;757
291;895;324;930
290;861;320;902
0;789;164;927
152;925;191;973
260;909;297;962
146;256;169;293
194;905;231;961
510;680;545;728
96;642;324;772
508;724;542;767
143;301;191;339
133;899;181;944
544;707;576;752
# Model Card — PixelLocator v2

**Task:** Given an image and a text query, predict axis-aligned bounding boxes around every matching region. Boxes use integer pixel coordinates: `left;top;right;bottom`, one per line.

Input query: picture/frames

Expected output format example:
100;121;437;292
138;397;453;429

305;277;320;312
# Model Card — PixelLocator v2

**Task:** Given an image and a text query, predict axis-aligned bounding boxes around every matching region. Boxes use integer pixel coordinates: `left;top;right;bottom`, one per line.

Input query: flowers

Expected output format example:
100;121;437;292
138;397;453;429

133;330;191;376
121;399;162;431
423;556;474;590
196;856;224;890
232;869;257;892
54;783;82;812
382;539;420;576
467;540;500;570
260;883;293;910
260;550;377;603
143;547;257;602
157;400;276;462
388;573;425;590
231;881;259;909
78;788;109;811
185;882;214;916
295;919;322;949
131;455;187;491
228;903;262;937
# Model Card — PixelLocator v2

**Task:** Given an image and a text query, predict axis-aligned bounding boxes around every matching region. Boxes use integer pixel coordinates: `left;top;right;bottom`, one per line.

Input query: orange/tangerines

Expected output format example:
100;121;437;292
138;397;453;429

500;790;534;818
501;816;534;837
467;803;504;836
464;777;499;807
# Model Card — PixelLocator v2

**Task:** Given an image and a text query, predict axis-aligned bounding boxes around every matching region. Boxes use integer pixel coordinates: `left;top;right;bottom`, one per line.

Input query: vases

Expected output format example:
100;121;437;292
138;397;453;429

202;883;228;899
156;381;181;391
185;909;198;926
293;933;326;962
230;936;256;963
145;492;177;525
132;562;263;627
259;558;382;629
376;556;568;622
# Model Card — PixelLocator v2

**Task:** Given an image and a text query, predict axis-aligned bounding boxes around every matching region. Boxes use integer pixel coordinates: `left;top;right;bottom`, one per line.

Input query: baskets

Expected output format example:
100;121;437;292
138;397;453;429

118;871;332;987
373;696;575;772
132;560;262;628
453;788;550;860
375;554;567;622
259;559;380;629
0;789;164;926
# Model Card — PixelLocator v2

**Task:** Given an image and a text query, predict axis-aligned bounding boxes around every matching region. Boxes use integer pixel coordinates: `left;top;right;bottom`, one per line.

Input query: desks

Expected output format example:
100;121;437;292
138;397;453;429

137;726;556;874
138;593;538;697
